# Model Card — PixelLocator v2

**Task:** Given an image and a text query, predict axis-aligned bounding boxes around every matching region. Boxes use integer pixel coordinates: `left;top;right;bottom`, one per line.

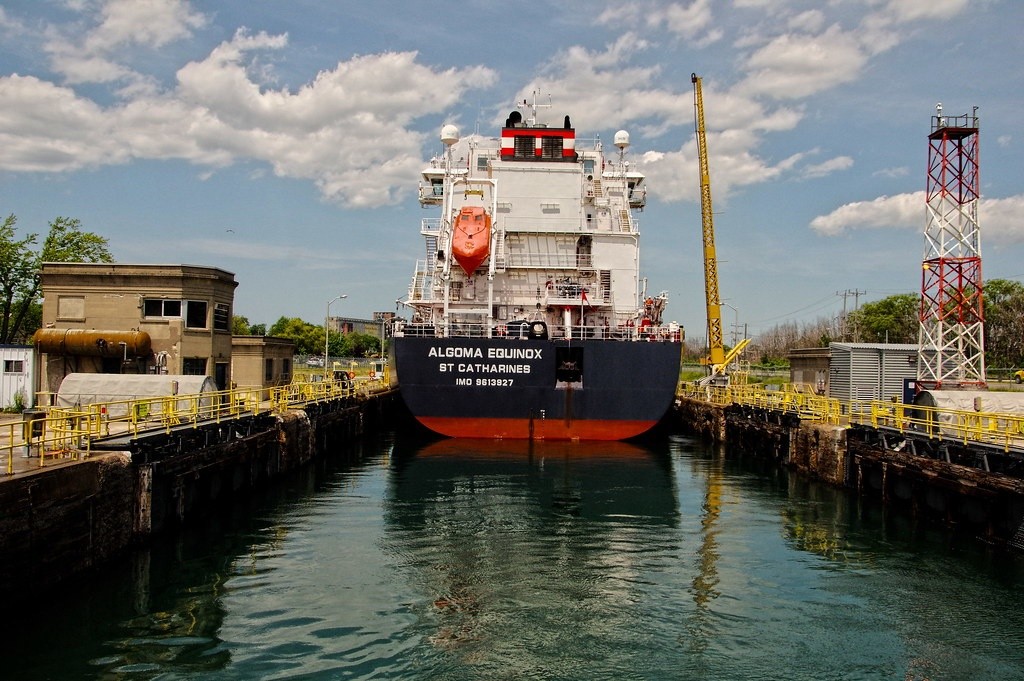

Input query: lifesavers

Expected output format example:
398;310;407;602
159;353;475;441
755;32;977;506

348;372;355;379
369;371;375;377
530;321;547;335
497;324;507;335
659;328;670;337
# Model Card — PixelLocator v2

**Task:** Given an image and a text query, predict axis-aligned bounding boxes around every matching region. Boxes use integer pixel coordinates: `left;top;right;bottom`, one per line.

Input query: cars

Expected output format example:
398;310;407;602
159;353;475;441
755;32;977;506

1014;369;1024;384
319;359;325;363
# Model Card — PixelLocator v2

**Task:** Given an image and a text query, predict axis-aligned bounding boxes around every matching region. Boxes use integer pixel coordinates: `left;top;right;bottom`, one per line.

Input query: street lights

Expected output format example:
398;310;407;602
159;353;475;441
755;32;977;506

324;294;347;379
720;302;739;385
381;317;397;374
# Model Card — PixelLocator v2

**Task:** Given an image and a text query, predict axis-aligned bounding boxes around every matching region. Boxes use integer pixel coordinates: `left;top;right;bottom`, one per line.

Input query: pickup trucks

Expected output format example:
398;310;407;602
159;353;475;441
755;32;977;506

307;357;324;368
322;370;354;392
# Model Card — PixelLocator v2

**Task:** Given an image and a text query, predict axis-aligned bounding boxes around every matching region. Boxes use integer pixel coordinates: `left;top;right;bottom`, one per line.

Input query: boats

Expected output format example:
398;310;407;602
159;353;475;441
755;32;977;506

391;87;685;443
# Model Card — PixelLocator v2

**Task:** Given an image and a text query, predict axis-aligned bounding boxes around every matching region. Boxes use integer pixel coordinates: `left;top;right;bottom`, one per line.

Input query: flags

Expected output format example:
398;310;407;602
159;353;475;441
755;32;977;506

582;289;592;308
